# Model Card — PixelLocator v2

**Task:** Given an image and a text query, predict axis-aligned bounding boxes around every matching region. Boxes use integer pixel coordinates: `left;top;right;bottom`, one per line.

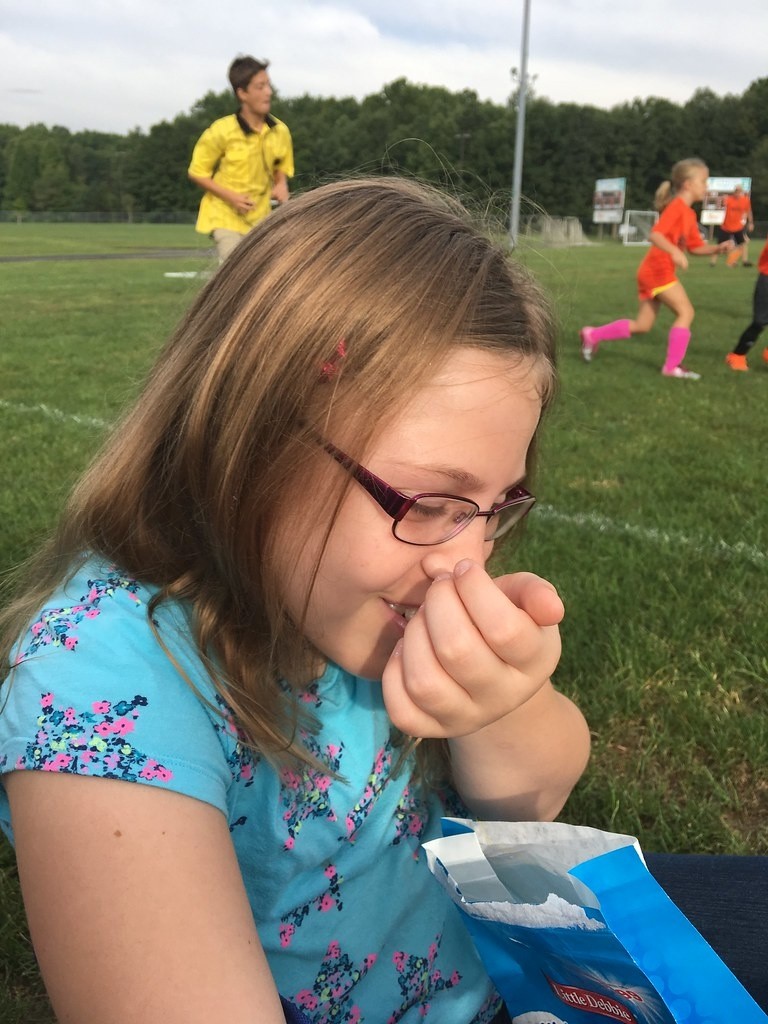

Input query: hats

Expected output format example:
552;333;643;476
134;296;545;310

228;57;269;90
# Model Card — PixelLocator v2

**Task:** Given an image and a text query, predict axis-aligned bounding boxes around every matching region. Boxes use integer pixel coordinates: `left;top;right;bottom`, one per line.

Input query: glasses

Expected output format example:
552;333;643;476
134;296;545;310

298;420;535;546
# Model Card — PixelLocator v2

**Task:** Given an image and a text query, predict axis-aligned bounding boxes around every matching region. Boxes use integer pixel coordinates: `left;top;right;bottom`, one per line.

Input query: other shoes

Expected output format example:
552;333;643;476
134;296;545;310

762;348;768;363
661;365;700;381
726;353;749;371
579;327;594;363
742;262;753;267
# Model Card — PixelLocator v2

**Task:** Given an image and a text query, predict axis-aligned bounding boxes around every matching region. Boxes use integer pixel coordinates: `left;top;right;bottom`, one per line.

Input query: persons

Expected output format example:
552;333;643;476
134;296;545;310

185;54;298;271
2;175;766;1022
709;182;755;268
578;157;735;379
723;242;768;371
